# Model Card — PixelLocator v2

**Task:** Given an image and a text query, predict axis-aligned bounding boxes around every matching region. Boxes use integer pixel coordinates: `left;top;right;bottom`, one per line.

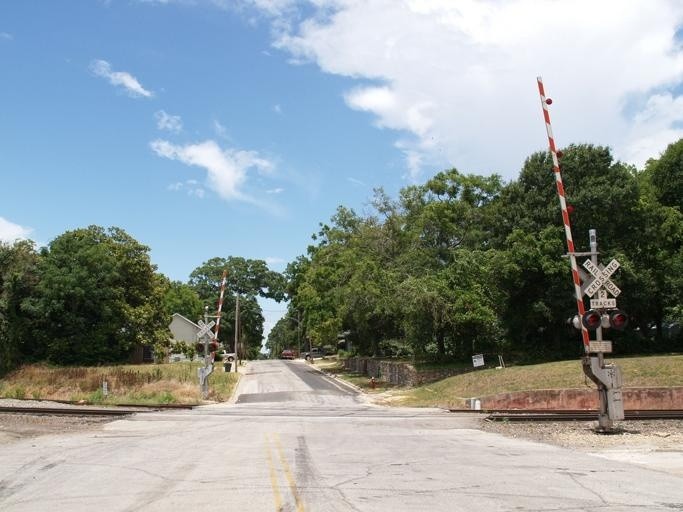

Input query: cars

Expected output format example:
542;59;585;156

281;349;297;359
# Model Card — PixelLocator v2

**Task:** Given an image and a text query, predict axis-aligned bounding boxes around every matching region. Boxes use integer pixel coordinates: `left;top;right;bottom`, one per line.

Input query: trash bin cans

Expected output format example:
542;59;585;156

224;363;231;372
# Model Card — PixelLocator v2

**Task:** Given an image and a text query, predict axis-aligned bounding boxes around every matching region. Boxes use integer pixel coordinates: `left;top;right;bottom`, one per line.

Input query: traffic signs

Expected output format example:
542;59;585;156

582;258;622;298
589;298;617;309
197;320;216;339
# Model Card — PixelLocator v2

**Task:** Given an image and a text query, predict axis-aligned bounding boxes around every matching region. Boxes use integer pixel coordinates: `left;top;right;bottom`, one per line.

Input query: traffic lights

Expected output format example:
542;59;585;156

208;342;218;352
609;310;627;328
196;343;204;353
581;309;601;329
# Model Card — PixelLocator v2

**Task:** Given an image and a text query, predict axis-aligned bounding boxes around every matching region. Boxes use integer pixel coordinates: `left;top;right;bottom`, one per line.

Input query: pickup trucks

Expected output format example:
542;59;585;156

216;350;237;362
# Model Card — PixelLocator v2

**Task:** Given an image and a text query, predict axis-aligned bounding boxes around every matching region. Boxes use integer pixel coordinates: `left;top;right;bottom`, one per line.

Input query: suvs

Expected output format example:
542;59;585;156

302;347;325;360
